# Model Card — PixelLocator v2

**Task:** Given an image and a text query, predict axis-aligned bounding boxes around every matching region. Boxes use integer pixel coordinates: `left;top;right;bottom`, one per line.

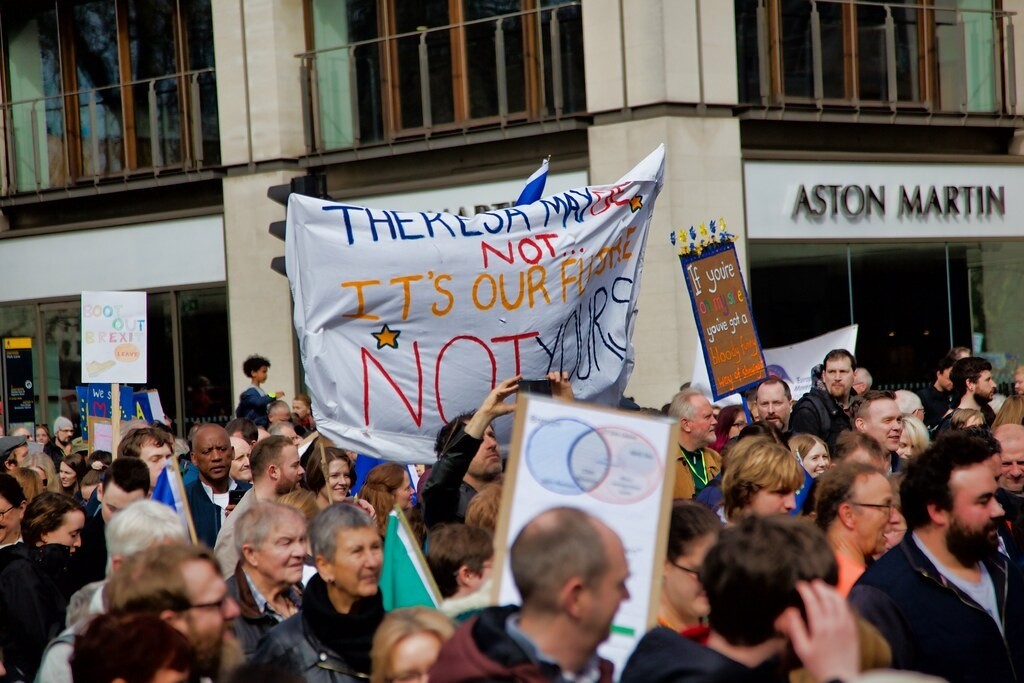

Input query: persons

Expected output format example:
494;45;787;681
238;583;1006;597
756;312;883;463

0;347;1024;683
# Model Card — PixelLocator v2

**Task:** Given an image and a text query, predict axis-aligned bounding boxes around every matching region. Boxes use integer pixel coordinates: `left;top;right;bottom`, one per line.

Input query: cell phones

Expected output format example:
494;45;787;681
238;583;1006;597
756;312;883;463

516;378;552;400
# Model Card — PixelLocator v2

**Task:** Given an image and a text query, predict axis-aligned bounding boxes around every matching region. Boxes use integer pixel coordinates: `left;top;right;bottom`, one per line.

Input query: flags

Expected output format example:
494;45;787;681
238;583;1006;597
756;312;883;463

516;160;553;206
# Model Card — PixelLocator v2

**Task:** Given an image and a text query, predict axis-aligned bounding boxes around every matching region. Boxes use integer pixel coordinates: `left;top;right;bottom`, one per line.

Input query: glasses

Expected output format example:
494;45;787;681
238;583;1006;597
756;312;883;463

852;504;902;517
732;422;746;430
0;506;14;521
171;596;226;611
669;560;704;583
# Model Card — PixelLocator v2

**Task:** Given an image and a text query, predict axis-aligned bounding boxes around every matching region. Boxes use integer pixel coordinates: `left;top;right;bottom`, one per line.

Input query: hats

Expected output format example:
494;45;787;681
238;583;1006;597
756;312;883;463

0;434;26;455
54;416;73;433
